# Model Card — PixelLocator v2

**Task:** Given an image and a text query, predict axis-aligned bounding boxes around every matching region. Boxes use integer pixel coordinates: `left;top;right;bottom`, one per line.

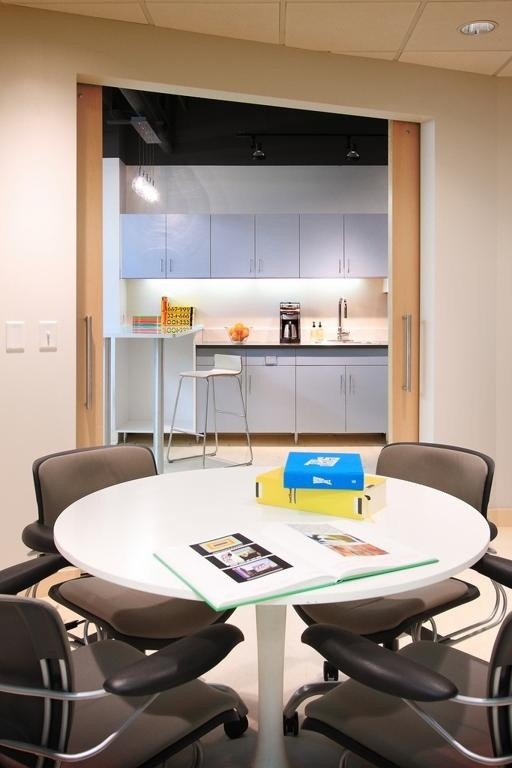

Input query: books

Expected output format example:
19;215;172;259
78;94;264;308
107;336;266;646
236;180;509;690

155;520;433;611
132;295;196;334
282;450;363;489
255;467;387;520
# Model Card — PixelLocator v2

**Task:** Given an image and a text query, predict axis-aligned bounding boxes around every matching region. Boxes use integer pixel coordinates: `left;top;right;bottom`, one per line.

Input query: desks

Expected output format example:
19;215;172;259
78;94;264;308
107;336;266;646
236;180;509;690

115;326;204;444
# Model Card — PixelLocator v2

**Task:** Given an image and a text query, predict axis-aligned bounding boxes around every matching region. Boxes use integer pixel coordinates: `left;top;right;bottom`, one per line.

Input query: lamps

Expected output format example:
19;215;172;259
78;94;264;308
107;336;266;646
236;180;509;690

235;132;388;162
129;116;160;203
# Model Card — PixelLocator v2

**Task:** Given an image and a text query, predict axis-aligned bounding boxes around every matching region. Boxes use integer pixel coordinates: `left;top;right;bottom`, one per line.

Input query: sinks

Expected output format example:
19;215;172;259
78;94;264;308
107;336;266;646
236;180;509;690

321;341;374;345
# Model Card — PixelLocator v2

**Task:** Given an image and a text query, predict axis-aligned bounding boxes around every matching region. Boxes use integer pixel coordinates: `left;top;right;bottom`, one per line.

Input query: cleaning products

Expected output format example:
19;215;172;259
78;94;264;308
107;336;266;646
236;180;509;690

317;319;324;339
310;320;317;340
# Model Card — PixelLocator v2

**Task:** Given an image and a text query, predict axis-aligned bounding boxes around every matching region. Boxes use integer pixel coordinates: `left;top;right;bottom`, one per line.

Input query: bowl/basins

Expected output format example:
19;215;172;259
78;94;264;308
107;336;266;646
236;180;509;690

223;326;254;343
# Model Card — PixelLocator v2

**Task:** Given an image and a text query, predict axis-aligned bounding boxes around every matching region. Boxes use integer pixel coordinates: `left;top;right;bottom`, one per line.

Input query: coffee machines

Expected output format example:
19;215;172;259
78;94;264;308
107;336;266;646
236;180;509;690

279;301;301;346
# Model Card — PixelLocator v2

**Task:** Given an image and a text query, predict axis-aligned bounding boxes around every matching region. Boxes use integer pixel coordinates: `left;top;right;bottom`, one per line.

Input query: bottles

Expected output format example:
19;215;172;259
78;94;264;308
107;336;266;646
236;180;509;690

310;321;325;342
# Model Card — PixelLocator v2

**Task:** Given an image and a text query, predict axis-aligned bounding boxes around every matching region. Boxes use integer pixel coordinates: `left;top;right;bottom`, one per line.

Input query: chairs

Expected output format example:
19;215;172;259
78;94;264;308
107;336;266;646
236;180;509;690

166;354;253;469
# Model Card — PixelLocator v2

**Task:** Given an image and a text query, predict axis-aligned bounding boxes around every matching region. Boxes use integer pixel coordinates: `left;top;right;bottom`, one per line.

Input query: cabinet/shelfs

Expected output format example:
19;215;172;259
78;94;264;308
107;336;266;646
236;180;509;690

211;214;300;279
196;347;296;444
119;214;211;279
300;213;388;279
296;347;390;443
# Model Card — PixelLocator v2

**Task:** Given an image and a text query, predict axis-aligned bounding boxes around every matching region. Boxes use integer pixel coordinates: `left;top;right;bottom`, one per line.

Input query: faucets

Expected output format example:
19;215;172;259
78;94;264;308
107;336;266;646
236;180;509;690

337;293;349;336
